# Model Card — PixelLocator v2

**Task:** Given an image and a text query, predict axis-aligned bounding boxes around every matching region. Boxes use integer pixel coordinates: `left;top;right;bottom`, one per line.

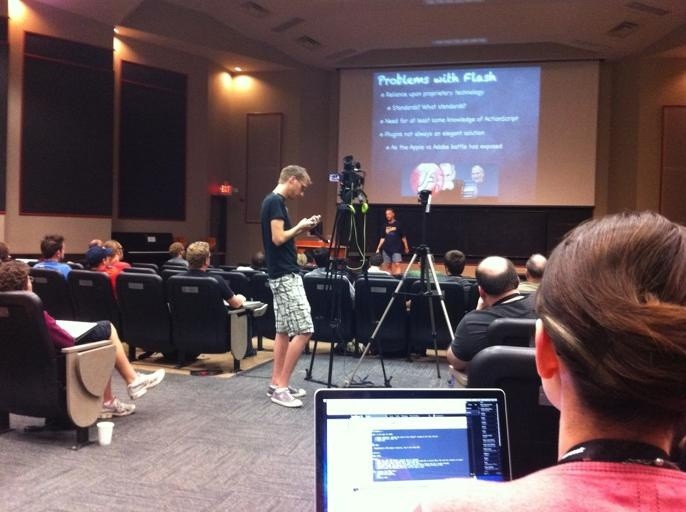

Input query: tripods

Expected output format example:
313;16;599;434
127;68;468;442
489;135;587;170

345;243;455;387
305;203;392;389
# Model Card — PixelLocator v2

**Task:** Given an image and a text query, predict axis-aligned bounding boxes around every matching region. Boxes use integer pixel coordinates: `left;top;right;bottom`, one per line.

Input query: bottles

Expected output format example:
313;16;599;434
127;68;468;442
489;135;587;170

459;179;479;199
447;365;455;387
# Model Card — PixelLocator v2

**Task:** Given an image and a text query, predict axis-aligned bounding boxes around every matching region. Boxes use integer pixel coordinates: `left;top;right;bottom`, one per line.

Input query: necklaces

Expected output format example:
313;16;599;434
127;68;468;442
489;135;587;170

557;459;685;471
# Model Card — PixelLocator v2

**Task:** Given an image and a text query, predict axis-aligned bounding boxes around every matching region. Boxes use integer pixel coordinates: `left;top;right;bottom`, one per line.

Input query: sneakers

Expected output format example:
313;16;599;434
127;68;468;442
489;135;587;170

266;384;306;407
101;395;136;419
248;304;269;318
127;368;165;400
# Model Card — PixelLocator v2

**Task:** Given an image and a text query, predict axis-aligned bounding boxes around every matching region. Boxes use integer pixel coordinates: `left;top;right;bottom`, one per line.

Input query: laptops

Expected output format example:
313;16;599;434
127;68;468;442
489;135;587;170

313;388;513;512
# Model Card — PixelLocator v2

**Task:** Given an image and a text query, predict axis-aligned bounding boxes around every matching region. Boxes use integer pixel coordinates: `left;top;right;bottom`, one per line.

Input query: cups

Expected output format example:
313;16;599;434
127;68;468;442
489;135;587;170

96;421;114;445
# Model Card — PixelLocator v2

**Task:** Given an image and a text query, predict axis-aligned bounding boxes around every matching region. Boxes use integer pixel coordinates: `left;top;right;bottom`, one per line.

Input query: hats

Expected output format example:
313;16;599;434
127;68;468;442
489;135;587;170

86;246;112;265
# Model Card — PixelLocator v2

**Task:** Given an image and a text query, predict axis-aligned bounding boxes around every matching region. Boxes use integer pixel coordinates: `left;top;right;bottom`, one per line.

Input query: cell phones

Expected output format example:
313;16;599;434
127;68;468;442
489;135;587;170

308;215;321;221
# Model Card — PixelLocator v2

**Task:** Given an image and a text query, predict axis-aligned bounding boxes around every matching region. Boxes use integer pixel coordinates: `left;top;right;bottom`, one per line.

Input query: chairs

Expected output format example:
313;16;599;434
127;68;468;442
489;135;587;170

0;239;270;371
450;317;560;482
270;239;481;356
1;291;119;450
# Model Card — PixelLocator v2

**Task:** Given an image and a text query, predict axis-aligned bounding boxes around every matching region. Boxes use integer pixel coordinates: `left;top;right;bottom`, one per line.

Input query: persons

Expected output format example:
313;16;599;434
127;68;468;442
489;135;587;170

461;165;499;196
376;208;409;275
260;164;322;408
413;212;686;511
